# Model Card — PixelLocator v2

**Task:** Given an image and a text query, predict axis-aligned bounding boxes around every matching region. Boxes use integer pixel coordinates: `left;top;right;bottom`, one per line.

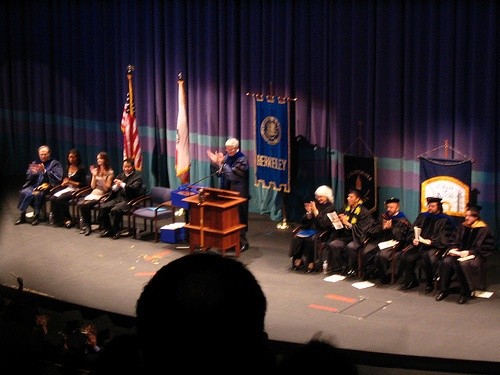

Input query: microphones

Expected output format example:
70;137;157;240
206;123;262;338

190;170;218;185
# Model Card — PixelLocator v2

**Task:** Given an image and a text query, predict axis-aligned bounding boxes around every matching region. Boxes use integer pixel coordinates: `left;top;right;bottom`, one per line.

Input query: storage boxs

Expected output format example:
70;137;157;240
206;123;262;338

173;221;187;241
178;184;208;194
170;188;198;209
159;225;181;243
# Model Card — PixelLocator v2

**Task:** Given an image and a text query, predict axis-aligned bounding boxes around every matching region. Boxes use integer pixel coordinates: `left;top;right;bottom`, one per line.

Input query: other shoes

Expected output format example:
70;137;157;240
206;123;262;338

31;216;39;225
14;215;26;225
306;265;315;274
330;263;342;272
102;230;111;237
80;223;91;236
65;219;72;228
292;259;303;271
381;271;388;282
112;228;120;240
342;266;352;276
363;271;373;281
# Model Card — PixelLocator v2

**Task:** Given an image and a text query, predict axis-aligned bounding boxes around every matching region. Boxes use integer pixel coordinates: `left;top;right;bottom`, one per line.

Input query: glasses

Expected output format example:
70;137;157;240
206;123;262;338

38;151;50;157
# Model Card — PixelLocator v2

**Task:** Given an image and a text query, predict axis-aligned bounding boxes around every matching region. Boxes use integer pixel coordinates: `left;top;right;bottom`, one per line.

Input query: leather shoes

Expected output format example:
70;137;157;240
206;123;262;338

425;283;434;293
239;243;248;252
401;279;418;291
436;289;448;301
459;293;469;303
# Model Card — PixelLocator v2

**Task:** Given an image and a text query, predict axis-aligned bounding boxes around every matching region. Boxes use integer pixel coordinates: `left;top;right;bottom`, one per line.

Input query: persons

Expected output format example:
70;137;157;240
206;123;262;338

78;152;115;235
360;197;411;285
207;137;250;253
99;158;143;239
400;197;455;294
48;148;87;228
0;254;357;375
325;188;371;276
289;185;337;274
14;145;64;226
436;205;495;305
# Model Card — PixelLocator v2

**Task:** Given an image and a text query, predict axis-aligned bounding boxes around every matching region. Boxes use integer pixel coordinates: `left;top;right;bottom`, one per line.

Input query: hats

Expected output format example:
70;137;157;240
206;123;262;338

348;188;361;199
466;202;482;214
427;196;442;204
385;198;399;204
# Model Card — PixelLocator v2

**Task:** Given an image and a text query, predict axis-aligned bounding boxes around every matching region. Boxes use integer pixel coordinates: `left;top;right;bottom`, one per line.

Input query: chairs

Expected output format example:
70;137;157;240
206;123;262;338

103;183;150;236
289;223;328;263
322;238;488;298
49;176;91;223
23;172;66;221
132;186;172;239
76;182;113;232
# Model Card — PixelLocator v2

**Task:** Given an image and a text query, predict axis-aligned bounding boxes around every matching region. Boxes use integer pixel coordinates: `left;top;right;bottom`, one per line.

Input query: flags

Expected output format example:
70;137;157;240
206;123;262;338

120;75;144;171
174;80;191;184
